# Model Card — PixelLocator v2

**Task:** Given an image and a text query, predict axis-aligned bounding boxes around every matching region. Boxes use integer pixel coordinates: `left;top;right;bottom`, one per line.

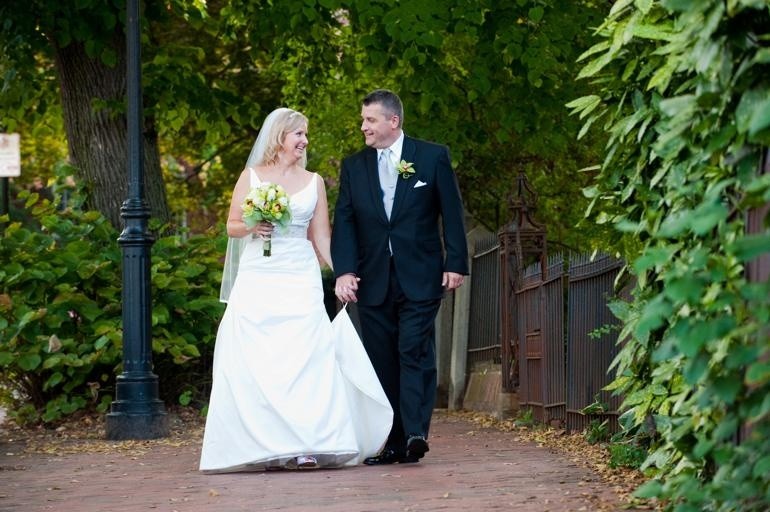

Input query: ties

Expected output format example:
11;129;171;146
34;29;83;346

381;149;396;256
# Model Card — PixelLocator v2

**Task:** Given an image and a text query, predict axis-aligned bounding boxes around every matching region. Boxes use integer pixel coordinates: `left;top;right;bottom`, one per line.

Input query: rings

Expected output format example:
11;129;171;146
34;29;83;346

457;283;462;286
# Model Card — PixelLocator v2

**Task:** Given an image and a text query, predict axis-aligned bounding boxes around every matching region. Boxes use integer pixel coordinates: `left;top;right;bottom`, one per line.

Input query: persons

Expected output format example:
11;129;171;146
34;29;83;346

198;106;395;474
330;89;469;467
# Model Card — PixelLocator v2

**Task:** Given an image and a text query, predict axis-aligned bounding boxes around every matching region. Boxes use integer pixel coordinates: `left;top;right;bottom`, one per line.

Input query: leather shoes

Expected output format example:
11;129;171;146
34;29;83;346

406;439;429;462
363;448;408;465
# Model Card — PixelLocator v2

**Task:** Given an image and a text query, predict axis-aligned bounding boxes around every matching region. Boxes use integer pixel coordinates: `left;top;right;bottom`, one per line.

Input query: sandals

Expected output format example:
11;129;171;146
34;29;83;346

297;457;317;466
